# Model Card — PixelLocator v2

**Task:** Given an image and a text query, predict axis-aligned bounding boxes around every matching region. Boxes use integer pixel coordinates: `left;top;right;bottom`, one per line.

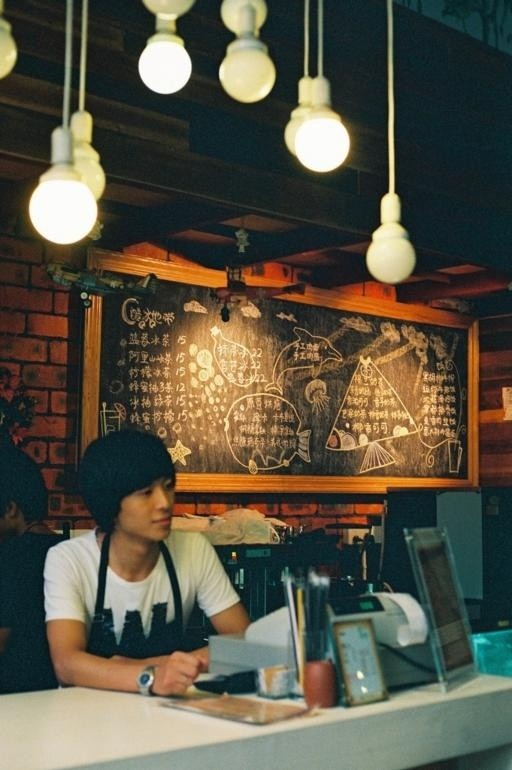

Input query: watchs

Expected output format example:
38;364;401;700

138;663;162;696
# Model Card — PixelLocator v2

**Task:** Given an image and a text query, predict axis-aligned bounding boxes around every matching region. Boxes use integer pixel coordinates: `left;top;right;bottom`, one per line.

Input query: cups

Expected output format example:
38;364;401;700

285;628;326;702
301;660;337;708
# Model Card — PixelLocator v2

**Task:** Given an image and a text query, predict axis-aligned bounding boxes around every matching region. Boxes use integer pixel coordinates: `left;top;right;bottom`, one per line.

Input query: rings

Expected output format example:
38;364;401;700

181;674;189;684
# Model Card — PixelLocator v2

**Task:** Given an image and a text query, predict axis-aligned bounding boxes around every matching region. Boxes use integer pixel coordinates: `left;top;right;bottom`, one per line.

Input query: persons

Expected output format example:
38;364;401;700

1;447;70;695
43;429;255;698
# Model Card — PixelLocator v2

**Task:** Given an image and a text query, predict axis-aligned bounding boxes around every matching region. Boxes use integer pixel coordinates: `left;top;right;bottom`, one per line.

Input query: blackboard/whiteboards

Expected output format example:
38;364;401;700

79;245;480;493
